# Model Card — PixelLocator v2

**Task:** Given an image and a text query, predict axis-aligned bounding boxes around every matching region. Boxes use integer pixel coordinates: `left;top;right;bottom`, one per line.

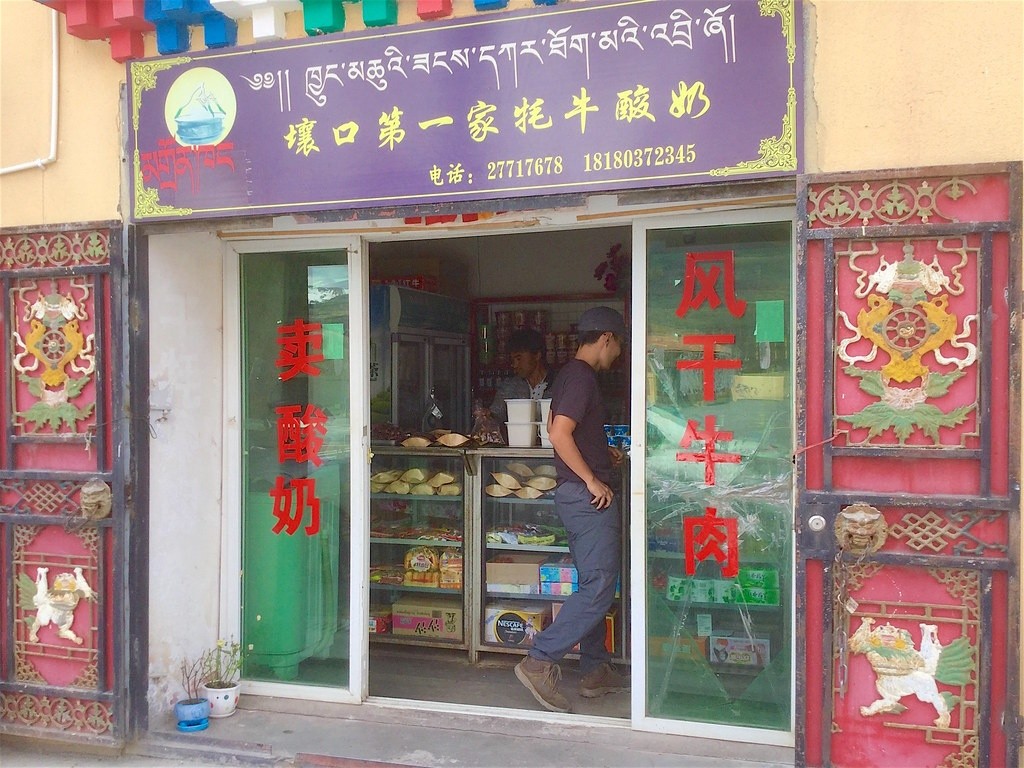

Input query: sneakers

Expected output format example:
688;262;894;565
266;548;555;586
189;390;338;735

580;660;631;697
514;656;572;713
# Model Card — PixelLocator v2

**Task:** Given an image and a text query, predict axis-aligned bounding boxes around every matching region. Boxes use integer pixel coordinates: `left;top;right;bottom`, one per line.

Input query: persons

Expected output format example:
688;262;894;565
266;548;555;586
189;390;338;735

514;307;631;711
489;330;554;422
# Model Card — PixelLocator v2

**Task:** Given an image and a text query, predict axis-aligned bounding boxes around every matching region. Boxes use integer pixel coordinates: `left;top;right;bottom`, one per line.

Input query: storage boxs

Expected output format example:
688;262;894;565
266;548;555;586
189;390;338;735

486;552;540;594
539;399;552;421
710;637;771;667
502;423;538;447
552;603;614;652
644;639;705;659
537;424;553;448
392;592;463;642
485;601;541;644
504;399;538;422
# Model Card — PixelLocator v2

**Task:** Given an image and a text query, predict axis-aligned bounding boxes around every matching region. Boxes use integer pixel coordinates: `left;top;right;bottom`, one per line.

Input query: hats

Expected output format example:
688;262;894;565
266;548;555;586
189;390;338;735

576;306;630;336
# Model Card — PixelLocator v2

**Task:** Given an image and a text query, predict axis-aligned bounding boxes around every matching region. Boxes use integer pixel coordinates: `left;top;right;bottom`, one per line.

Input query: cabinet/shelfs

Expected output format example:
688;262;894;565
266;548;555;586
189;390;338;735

373;450;628;666
479;297;630;420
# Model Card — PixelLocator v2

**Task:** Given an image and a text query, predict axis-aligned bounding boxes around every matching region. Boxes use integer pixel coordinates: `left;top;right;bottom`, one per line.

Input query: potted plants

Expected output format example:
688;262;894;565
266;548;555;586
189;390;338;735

176;659;209;731
199;638;241;718
